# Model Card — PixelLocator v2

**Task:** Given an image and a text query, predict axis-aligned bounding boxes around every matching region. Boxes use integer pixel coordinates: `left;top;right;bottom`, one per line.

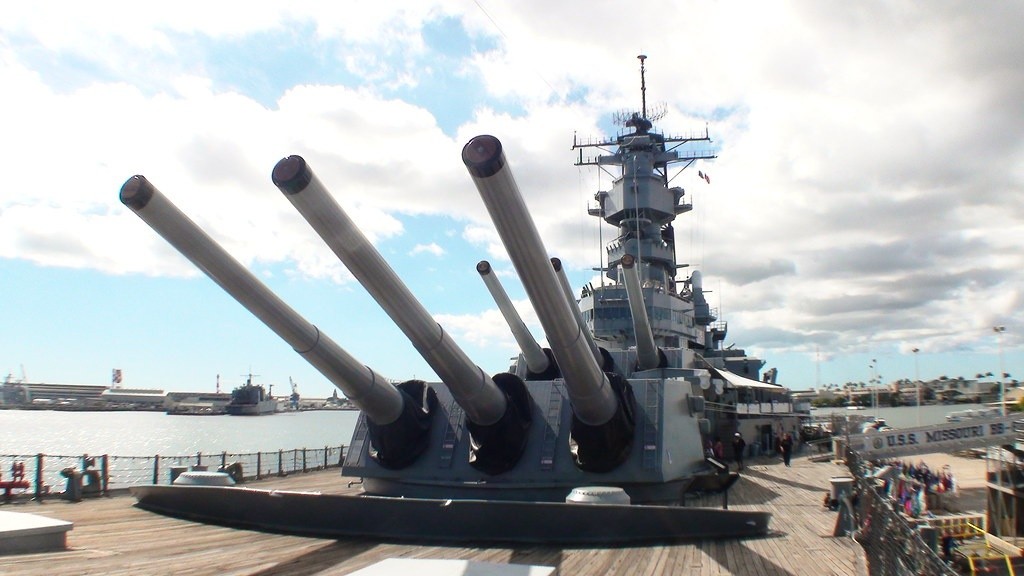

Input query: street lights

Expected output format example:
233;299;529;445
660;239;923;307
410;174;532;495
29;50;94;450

992;326;1007;416
912;348;920;405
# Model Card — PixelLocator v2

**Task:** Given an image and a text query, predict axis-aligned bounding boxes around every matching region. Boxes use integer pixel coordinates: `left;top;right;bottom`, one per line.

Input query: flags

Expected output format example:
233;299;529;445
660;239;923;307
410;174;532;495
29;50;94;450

699;171;704;179
705;174;710;184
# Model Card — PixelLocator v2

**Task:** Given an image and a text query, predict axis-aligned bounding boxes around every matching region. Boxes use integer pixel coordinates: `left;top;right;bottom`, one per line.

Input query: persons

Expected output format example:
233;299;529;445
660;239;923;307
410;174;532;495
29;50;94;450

704;440;714;458
732;433;745;470
780;433;793;467
713;437;723;459
818;424;824;438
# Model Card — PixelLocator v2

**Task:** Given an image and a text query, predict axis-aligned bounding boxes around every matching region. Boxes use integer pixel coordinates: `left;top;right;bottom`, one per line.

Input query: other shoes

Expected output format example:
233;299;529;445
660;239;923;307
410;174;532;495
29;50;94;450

739;468;741;470
788;463;789;465
742;468;744;470
785;464;788;467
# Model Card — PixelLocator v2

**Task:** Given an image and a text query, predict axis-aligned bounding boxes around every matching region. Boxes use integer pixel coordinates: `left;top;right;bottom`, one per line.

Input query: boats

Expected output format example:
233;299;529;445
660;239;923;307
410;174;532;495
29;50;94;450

224;374;278;415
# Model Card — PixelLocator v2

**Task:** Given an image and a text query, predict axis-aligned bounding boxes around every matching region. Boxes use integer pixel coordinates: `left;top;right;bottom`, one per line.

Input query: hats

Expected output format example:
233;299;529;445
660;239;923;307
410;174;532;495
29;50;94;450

735;433;741;438
